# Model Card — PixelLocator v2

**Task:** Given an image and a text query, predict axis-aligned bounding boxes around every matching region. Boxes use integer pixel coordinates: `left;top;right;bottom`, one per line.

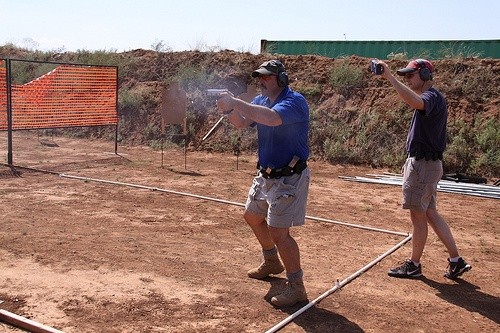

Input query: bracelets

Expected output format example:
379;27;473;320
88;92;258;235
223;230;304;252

223;109;233;115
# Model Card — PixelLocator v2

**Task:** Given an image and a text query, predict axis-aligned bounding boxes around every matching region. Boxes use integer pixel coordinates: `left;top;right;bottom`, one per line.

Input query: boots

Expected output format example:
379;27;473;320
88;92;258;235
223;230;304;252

271;280;307;307
247;255;285;277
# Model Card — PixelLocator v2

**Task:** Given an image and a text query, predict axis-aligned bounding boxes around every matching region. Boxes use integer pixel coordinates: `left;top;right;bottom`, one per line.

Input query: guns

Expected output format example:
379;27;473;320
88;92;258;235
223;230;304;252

205;88;235;115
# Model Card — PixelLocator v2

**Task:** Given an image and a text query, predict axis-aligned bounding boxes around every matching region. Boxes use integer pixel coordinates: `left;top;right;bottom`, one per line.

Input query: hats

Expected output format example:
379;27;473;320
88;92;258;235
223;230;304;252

252;60;286;78
397;59;434;76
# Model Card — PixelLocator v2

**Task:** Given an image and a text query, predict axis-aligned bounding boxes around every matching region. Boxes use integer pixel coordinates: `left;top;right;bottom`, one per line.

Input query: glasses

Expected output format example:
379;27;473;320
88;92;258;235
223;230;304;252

404;71;419;79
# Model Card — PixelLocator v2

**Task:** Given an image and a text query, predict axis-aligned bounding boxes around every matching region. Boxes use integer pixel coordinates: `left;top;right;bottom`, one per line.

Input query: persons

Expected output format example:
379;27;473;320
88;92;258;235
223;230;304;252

216;60;309;306
371;59;472;279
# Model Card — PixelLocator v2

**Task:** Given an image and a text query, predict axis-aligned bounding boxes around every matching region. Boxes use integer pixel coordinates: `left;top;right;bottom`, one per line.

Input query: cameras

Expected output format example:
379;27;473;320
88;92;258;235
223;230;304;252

369;59;381;75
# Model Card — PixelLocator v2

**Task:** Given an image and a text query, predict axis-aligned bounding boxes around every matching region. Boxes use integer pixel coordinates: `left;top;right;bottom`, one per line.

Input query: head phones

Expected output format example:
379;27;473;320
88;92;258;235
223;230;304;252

415;59;432;81
272;60;289;88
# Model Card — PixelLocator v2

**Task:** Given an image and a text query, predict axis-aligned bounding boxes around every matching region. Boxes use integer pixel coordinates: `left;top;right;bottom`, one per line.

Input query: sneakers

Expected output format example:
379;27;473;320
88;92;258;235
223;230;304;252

445;257;472;280
388;259;422;278
